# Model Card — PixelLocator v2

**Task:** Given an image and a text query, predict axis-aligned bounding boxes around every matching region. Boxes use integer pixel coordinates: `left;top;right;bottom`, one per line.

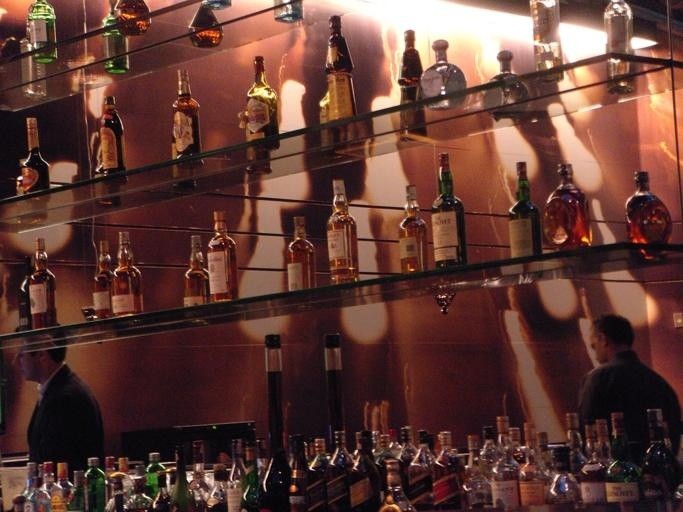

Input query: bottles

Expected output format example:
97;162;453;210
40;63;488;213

400;30;426;140
543;163;595;255
173;67;204;190
16;117;50;223
623;170;670;259
423;38;467;113
18;209;315;339
187;1;223;47
96;96;125;208
2;408;682;512
487;51;528;121
102;0;151;75
396;185;428;280
327;177;359;287
322;85;345;157
323;15;359;122
528;1;565;83
243;55;279;175
600;1;638;95
430;151;469;268
506;161;543;258
27;0;58;64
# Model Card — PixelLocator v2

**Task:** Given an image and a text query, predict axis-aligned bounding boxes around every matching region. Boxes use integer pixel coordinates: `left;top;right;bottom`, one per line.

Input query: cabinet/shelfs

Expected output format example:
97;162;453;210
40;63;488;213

0;0;683;360
121;422;256;465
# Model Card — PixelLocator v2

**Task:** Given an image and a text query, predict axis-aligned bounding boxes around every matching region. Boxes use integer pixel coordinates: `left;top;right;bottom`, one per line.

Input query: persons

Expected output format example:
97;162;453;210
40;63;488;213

11;324;106;485
577;312;681;457
